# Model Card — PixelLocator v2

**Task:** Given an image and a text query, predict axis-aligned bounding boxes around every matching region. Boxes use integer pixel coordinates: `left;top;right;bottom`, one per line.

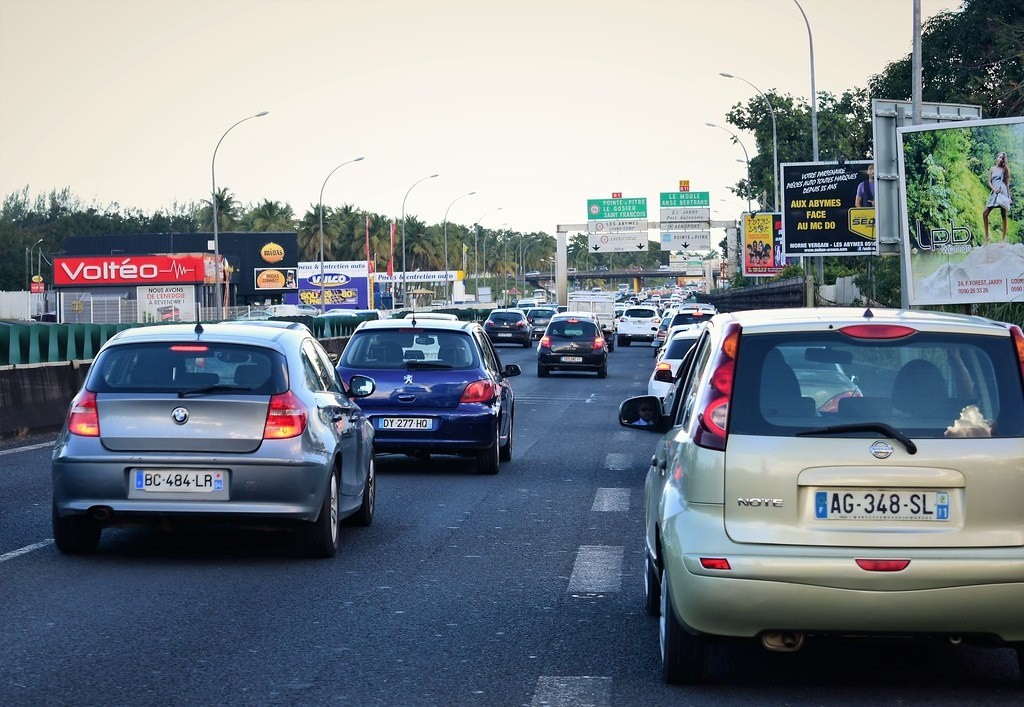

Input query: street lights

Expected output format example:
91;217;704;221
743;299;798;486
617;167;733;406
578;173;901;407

320;158;366;315
211;111;271;321
707;123;751;213
445;189;541;301
540;259;552;303
719;72;779;211
402;174;437;307
30;239;44;277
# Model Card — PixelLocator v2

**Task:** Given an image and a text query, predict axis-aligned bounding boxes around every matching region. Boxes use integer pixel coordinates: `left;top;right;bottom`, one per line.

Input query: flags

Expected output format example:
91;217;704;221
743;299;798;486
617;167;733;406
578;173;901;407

463;243;468;271
387;224;396;276
366;217;375;273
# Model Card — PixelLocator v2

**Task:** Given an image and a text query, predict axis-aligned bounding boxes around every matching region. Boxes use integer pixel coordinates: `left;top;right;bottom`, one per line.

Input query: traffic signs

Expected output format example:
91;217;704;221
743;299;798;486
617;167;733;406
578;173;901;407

660;232;710;250
588;232;648;253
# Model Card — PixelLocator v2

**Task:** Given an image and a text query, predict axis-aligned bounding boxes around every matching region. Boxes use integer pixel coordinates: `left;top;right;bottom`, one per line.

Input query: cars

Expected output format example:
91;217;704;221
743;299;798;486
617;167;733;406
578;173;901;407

616;286;863;417
336;307;608;474
238;305;379;320
49;320;377;557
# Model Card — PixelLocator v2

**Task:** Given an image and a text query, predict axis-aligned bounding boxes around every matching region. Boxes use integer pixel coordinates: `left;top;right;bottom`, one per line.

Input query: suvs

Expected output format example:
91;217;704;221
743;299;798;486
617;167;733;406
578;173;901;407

626;267;642;274
589;266;608;272
525;271;539;276
618;302;1024;684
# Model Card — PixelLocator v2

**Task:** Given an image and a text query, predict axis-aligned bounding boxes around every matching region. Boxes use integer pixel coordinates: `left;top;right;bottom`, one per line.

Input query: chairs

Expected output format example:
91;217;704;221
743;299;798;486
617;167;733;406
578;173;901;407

128;349;266;387
556;324;592;337
763;347;980;419
369;341;465;367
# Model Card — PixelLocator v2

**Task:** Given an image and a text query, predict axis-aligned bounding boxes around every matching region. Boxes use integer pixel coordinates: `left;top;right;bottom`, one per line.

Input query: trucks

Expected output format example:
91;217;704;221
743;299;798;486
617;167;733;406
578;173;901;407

567;293;616;353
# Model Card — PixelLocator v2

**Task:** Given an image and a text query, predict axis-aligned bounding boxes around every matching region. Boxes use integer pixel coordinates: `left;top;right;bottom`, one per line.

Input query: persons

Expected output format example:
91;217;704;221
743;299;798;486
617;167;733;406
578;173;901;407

746;240;771;265
893;350;981;418
982;152;1012;248
855;164;875;207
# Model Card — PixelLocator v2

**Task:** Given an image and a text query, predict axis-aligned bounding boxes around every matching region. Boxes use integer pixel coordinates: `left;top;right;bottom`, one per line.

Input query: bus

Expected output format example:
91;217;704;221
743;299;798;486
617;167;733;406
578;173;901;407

532;289;546;304
